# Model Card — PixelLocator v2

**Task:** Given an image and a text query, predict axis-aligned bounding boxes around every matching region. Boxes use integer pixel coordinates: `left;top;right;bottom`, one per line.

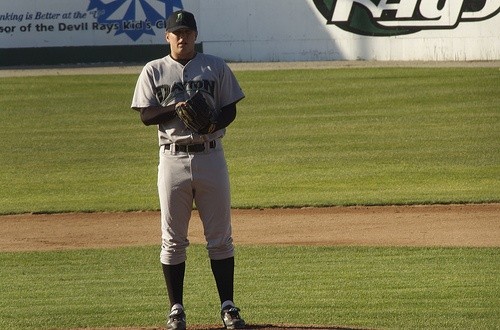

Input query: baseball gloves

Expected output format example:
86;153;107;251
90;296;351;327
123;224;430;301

176;91;215;134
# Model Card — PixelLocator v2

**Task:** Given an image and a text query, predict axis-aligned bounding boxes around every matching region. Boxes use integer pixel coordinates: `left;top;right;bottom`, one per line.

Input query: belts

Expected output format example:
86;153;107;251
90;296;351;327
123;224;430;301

165;141;216;152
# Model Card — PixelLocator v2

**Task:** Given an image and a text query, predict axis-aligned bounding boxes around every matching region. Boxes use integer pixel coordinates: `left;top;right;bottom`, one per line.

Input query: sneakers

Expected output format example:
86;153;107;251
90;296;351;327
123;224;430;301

221;307;245;330
167;308;186;330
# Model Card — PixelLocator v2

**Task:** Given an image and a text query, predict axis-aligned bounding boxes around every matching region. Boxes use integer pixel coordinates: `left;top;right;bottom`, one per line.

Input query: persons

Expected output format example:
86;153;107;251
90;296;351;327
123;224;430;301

130;9;246;330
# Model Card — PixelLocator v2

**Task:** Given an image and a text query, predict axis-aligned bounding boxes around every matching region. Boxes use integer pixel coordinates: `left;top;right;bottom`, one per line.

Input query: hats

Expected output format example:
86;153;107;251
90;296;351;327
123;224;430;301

166;10;197;33
